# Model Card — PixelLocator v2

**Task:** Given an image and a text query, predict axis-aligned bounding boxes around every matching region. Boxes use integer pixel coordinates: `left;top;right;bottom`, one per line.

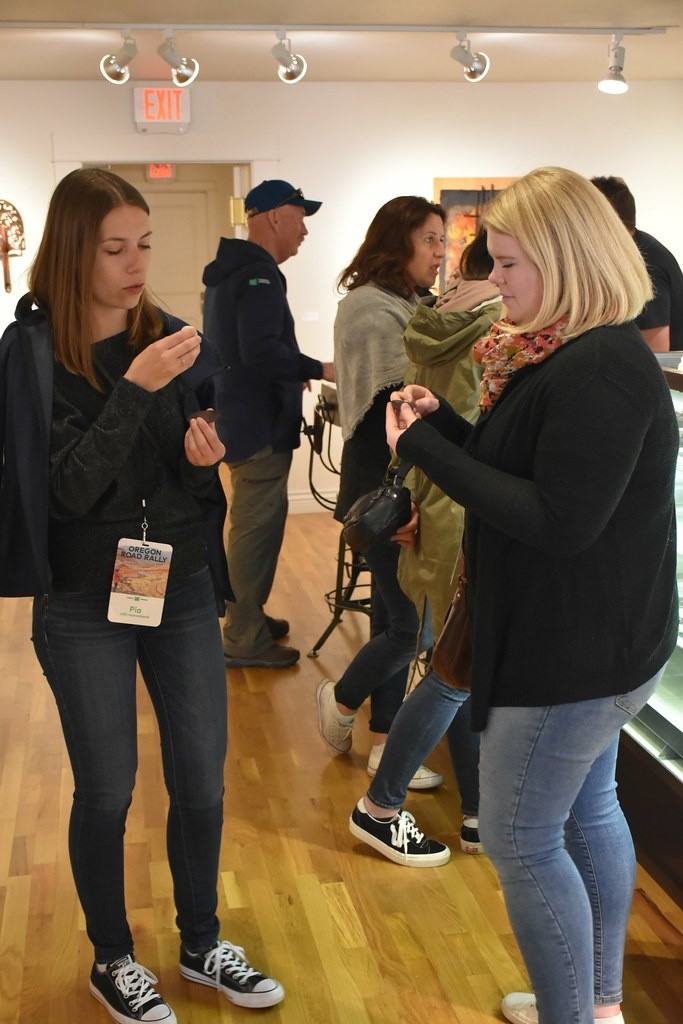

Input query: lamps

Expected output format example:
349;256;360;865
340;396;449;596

156;30;200;88
450;32;491;82
597;33;629;95
0;199;26;295
272;31;307;83
99;29;137;85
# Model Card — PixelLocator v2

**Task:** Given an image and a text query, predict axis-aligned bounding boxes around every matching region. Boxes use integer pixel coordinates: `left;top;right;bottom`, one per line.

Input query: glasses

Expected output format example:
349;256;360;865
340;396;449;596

275;187;305;211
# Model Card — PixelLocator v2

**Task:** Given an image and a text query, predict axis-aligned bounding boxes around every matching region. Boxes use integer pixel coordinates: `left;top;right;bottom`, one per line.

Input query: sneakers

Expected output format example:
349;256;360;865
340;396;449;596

501;992;627;1024
179;934;283;1008
88;954;179;1024
460;816;483;854
367;745;442;790
223;642;301;666
268;617;289;637
317;679;357;753
349;797;450;868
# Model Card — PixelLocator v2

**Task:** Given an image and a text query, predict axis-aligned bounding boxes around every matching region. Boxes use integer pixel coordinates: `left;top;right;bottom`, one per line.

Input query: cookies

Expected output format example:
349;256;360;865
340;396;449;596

186;411;221;423
391;399;416;410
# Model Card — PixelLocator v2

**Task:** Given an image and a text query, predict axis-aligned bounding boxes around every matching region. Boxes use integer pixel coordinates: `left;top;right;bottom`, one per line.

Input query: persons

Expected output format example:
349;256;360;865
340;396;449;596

201;181;336;668
387;165;683;1024
0;166;285;1024
316;195;497;869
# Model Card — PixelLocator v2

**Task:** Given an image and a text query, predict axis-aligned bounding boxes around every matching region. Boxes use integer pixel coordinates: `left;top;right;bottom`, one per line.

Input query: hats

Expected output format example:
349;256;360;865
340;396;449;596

243;181;323;222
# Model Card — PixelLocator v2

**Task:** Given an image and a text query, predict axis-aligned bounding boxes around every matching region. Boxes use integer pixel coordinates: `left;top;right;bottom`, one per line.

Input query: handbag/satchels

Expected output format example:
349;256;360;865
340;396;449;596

342;481;416;580
